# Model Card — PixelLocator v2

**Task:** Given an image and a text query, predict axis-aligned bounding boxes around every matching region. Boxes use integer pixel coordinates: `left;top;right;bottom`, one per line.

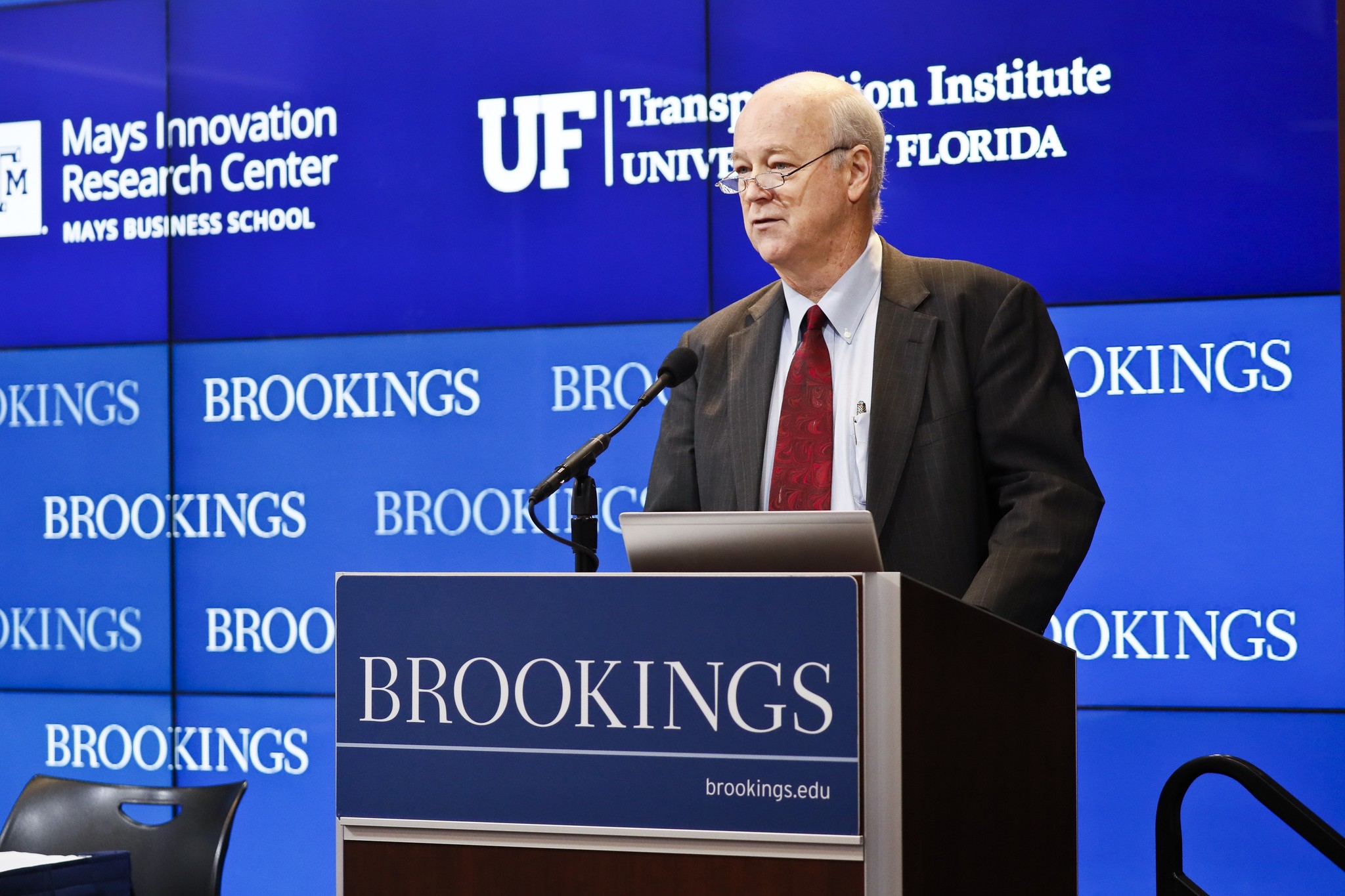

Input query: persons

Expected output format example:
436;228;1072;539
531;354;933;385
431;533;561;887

647;69;1104;635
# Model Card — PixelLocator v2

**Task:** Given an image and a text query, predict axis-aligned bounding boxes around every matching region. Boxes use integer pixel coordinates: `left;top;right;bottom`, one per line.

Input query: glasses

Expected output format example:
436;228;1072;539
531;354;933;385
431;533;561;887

715;147;853;195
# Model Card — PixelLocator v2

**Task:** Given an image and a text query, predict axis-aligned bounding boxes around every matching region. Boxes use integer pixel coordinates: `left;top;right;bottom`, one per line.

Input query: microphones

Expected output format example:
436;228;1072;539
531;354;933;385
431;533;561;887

637;347;698;407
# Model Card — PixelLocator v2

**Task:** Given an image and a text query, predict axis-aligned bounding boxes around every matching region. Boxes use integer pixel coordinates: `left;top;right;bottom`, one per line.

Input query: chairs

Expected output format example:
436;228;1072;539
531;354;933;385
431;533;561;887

0;771;249;896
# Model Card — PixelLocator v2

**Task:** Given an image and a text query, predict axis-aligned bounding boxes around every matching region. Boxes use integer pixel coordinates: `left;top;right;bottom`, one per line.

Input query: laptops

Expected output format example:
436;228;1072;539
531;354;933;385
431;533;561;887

617;511;884;571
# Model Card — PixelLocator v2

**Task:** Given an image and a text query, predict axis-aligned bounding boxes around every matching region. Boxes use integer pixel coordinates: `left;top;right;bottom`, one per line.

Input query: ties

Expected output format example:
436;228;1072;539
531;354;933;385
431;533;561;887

767;303;835;512
